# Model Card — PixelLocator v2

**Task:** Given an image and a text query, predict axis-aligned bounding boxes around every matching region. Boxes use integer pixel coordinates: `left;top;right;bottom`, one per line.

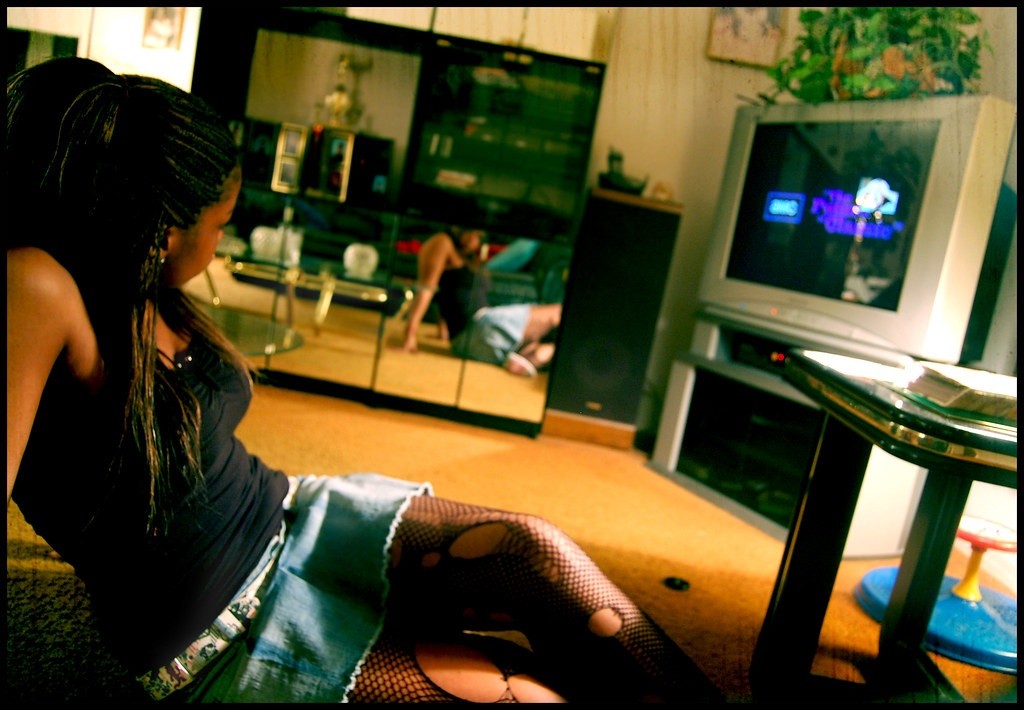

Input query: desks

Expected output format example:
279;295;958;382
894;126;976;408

751;348;1019;703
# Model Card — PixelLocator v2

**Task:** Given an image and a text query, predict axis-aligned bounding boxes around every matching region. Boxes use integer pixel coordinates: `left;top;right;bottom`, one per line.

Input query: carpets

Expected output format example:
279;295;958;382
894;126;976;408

7;497;1018;703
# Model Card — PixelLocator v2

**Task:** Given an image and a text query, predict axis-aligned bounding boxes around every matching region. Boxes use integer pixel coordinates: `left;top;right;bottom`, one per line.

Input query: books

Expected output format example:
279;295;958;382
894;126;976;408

909;359;1017;420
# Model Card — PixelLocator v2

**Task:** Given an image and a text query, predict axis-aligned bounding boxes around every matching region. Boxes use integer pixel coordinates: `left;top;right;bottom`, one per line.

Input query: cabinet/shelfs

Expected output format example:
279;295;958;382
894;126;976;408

644;305;931;553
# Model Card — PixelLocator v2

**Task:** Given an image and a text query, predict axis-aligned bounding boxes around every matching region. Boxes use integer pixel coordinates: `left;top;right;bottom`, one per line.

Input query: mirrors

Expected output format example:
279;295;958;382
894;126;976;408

183;187;573;424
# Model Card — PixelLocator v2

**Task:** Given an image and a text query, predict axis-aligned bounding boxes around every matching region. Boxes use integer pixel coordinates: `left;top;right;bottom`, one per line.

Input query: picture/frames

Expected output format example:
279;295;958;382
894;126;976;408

704;7;790;70
141;6;186;51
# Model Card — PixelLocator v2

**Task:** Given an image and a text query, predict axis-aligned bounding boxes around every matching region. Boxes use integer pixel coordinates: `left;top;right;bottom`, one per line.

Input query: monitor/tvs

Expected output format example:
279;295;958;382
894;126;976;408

694;94;1019;372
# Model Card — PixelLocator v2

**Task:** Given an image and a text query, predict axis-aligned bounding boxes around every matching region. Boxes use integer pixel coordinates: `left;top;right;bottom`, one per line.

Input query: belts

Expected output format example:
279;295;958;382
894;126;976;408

135;475;300;702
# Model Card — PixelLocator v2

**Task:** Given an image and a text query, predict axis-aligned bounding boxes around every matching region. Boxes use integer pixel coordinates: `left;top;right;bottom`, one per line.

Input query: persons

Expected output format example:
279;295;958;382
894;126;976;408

1;57;733;706
402;222;561;375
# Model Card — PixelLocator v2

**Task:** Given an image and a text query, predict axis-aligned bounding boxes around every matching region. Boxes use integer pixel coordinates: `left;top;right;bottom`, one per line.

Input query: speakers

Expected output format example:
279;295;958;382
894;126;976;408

542;186;682;450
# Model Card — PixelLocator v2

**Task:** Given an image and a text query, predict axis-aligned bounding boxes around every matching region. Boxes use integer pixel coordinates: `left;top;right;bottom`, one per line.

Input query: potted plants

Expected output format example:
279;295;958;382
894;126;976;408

737;6;995;120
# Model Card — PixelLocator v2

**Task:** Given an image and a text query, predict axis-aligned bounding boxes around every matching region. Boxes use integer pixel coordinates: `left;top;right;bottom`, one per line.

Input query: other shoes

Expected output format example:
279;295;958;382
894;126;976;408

504;350;537;376
